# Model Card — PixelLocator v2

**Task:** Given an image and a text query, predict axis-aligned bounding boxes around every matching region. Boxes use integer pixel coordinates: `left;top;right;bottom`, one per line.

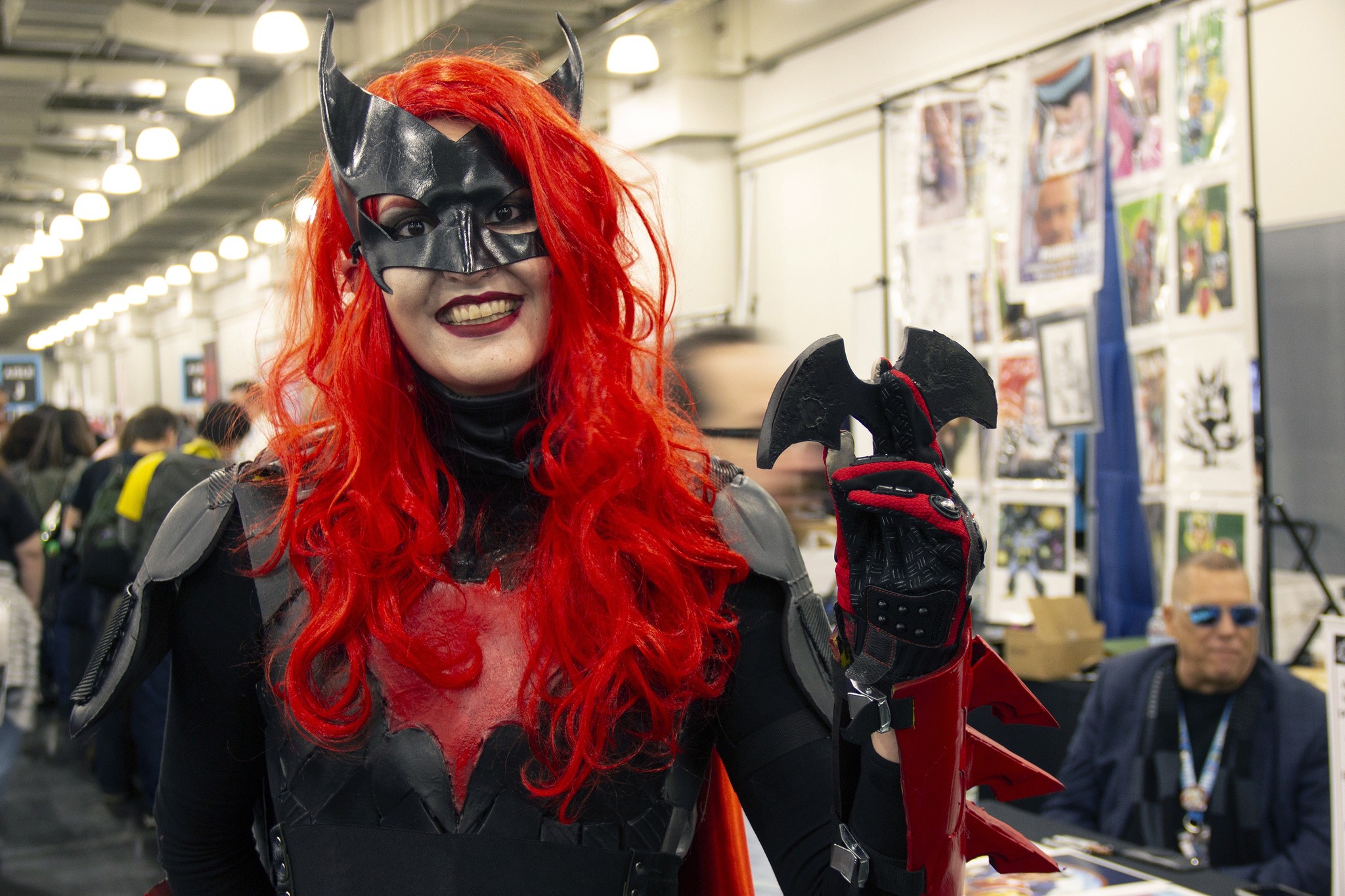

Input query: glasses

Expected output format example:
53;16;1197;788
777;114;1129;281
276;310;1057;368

1172;601;1264;628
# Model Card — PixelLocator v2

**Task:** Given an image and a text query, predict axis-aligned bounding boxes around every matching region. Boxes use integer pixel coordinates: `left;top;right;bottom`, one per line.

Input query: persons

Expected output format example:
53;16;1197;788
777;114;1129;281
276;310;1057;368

0;377;282;827
660;322;831;554
1044;547;1332;895
141;24;992;895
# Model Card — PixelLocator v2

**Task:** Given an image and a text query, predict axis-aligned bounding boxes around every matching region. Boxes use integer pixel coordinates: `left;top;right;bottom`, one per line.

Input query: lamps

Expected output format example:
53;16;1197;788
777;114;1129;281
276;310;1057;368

0;1;659;353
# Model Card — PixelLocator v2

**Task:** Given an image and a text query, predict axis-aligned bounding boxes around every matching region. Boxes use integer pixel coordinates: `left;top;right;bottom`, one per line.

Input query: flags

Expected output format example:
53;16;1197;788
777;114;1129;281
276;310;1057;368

1074;121;1156;639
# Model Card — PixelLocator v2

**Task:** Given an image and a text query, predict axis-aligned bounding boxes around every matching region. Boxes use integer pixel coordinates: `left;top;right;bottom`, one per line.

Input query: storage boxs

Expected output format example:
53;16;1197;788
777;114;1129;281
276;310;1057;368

1004;589;1104;685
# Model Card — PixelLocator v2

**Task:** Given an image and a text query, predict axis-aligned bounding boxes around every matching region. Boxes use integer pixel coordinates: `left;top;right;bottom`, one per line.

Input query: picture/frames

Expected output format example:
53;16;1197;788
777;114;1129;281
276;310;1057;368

1038;311;1101;432
